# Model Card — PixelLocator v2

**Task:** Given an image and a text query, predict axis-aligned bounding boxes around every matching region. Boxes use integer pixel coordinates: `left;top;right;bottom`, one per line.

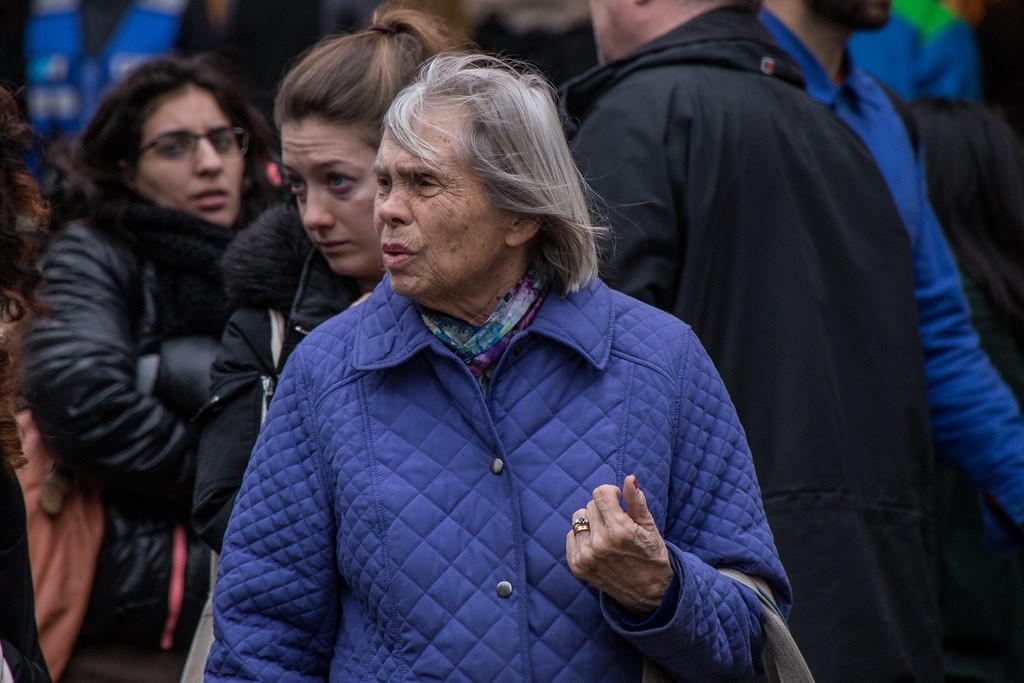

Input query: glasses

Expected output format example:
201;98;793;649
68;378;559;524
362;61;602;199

138;126;249;160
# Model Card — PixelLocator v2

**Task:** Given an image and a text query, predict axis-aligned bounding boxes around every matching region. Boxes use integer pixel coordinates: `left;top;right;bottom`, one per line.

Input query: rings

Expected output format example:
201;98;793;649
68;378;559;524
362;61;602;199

572;518;590;533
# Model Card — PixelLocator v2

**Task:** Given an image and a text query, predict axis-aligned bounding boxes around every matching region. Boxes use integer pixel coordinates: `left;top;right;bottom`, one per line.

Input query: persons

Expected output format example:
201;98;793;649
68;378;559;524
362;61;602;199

195;51;815;682
0;0;1024;683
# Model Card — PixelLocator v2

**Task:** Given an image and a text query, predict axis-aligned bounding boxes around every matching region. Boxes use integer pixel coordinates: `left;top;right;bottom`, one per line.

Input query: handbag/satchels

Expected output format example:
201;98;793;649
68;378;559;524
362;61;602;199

7;409;107;683
